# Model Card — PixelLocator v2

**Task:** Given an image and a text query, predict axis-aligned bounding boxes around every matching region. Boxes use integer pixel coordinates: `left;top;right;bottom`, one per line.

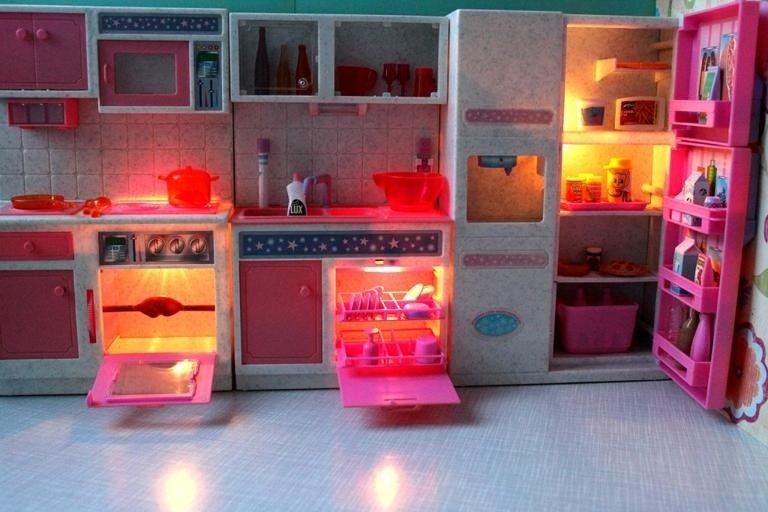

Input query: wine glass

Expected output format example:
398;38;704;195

398;64;409;96
383;63;396;92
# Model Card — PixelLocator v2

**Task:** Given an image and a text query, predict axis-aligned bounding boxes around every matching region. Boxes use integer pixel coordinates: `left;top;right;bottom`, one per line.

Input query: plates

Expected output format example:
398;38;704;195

418;285;434;301
604;260;647;276
337;286;384;322
400;284;423;301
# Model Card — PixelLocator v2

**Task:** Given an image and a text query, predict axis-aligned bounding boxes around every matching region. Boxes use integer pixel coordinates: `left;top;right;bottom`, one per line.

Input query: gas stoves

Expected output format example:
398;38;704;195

100;202;220;213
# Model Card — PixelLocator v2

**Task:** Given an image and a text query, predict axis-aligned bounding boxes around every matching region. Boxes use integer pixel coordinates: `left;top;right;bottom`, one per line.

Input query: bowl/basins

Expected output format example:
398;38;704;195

402;303;431;319
335;67;378;96
373;173;443;212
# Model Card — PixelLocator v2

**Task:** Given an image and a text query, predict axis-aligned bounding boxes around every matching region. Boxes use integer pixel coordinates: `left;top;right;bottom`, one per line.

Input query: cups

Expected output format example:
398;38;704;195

567;177;582;204
578;173;592;202
415;336;438;364
585;176;601;203
361;328;380;365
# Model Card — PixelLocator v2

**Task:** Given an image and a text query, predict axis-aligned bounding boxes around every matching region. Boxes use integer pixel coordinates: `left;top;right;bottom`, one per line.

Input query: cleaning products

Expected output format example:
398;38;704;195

287;173;307;216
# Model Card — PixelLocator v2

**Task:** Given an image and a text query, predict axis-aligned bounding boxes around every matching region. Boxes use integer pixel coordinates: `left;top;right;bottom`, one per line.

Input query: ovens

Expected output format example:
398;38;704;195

86;232;217;407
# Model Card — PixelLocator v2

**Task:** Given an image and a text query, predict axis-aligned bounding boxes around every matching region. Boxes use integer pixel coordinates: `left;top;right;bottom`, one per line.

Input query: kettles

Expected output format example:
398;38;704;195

603;158;631;202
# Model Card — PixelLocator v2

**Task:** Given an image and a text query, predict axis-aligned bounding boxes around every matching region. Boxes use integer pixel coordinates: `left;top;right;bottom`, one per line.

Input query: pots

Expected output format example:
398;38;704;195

10;195;72;209
158;166;219;208
